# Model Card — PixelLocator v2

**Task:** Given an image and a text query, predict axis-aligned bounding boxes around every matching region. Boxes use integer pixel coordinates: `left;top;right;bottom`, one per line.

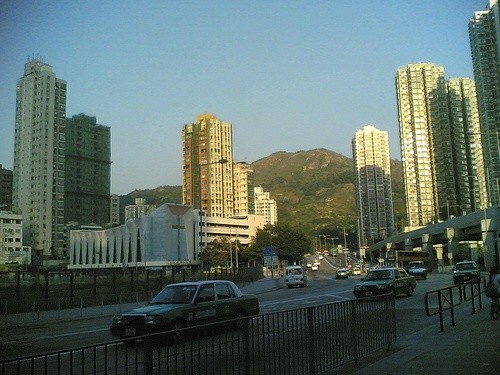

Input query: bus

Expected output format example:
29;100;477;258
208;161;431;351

385;250;432;272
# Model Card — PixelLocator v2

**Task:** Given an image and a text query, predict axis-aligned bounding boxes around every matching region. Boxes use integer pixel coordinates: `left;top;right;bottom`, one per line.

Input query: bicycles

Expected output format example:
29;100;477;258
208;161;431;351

490;294;500;320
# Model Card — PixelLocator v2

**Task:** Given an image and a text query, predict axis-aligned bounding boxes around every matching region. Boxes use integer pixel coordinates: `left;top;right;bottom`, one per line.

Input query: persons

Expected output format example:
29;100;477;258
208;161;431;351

486;265;500;319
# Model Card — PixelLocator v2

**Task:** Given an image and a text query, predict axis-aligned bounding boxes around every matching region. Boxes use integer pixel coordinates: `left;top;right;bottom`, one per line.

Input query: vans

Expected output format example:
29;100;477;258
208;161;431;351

285;265;308;289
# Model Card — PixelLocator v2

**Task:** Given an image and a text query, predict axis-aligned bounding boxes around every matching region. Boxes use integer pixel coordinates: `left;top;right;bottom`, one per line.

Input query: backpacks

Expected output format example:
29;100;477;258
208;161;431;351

486;273;500;297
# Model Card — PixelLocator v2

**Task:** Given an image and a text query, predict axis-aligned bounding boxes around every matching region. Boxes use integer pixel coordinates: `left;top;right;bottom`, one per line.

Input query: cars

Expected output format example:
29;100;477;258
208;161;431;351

353;267;417;302
451;260;481;286
408;261;427;280
110;280;260;347
306;248;338;271
336;245;379;280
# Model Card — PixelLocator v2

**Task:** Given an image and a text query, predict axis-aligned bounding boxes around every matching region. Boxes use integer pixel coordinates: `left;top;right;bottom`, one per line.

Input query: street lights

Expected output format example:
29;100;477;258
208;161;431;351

266;230;274;281
198;158;229;280
313;235;339;251
